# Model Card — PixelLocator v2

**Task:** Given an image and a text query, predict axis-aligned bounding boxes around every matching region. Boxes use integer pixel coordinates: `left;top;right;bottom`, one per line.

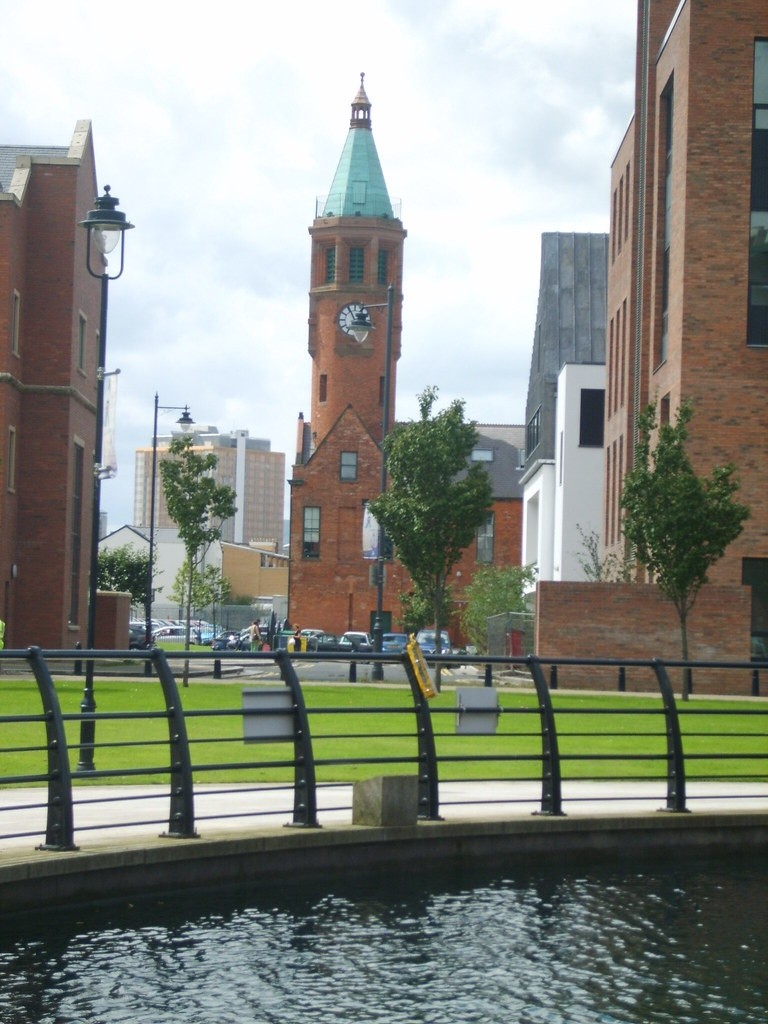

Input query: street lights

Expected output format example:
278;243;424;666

346;284;395;683
75;184;135;775
143;390;197;678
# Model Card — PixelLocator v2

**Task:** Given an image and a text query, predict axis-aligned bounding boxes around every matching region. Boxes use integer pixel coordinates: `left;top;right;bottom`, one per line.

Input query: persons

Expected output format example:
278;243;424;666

292;623;302;651
249;619;263;651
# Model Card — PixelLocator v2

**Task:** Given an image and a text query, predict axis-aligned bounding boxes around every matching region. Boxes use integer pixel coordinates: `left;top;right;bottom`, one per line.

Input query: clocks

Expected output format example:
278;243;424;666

339;303;370;336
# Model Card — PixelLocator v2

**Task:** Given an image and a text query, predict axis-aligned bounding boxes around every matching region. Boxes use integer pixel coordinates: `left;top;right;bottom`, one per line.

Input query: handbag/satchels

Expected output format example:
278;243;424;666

288;636;295;645
252;626;259;641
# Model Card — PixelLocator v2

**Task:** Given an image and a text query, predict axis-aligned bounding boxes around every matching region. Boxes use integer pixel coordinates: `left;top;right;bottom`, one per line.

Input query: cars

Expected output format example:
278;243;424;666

129;617;326;652
371;634;409;653
337;631;373;653
308;631;361;653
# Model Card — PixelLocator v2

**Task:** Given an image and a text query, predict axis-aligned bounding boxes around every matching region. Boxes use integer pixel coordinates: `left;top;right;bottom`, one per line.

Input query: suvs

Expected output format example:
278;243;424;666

416;628;453;654
